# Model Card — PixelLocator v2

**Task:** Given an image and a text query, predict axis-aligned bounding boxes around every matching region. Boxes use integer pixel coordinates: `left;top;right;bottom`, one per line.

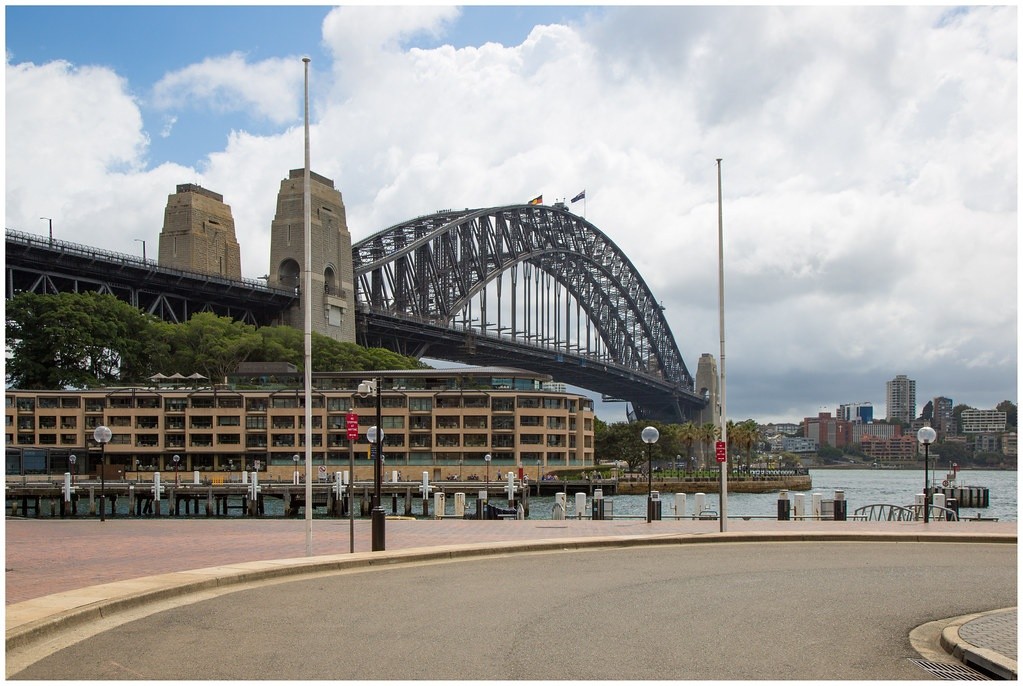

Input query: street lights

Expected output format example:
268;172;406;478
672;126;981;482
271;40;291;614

69;455;77;488
174;455;181;490
38;216;54;249
917;426;937;523
367;426;386;519
293;454;300;485
485;454;492;505
93;425;113;524
457;451;785;479
641;425;660;522
133;238;146;267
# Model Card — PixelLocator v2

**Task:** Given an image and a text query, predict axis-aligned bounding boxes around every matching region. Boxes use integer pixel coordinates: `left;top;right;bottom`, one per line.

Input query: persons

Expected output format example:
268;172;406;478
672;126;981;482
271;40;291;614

581;469;602;479
498;469;502;480
523;473;529;480
542;473;560;480
332;472;335;483
398;470;402;481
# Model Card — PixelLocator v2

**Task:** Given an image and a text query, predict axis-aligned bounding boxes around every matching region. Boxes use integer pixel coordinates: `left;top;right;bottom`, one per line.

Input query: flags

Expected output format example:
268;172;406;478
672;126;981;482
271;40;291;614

571;190;586;204
528;195;543;205
